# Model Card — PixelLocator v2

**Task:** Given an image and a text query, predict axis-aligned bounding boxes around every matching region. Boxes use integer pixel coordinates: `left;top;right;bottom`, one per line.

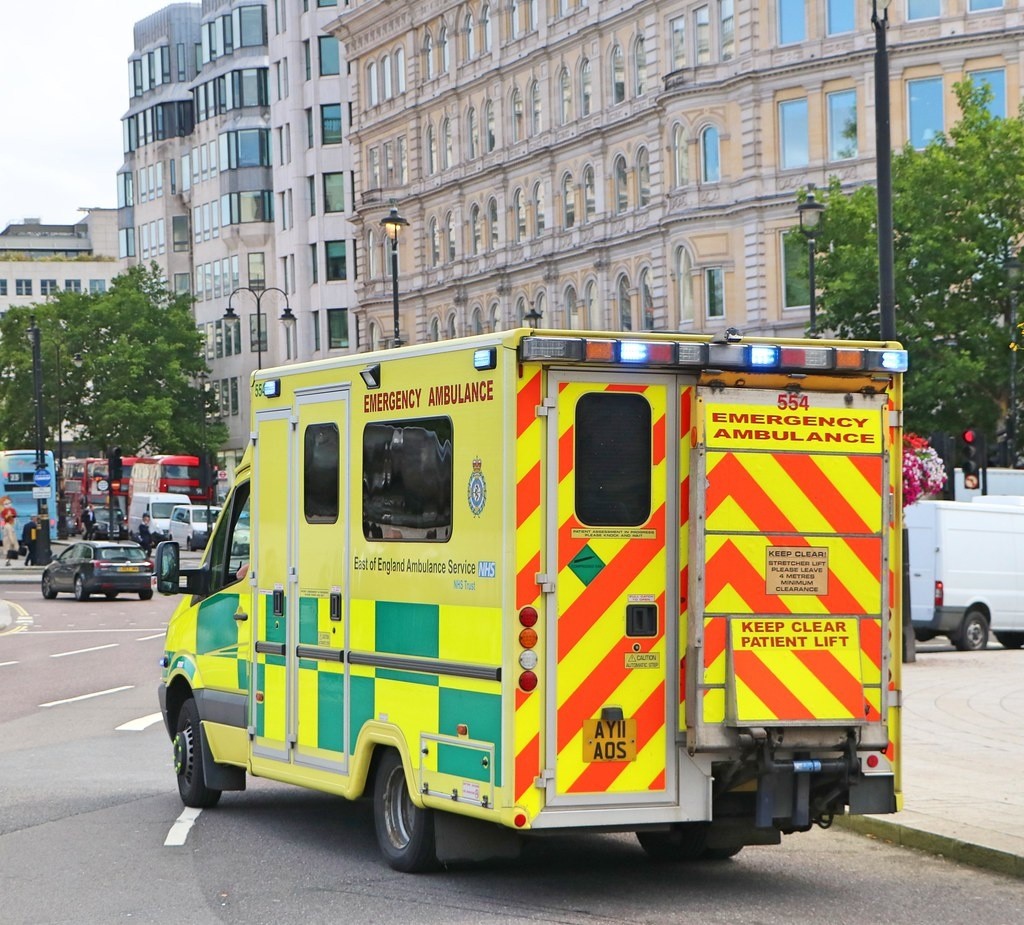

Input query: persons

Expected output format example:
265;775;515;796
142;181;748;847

22;514;36;566
81;504;96;540
236;562;248;578
3;517;20;565
138;513;172;546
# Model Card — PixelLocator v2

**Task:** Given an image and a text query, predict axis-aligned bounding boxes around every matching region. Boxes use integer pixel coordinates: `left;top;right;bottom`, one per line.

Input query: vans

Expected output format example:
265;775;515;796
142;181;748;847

128;493;192;547
904;500;1024;650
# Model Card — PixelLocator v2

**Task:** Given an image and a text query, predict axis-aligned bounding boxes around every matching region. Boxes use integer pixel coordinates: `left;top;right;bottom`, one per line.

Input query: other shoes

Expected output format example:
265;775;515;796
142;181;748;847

6;562;11;566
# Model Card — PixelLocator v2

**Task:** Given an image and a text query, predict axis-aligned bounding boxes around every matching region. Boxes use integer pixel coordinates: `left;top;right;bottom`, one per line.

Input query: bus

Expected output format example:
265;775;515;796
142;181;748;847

53;456;138;524
128;454;214;505
0;450;59;544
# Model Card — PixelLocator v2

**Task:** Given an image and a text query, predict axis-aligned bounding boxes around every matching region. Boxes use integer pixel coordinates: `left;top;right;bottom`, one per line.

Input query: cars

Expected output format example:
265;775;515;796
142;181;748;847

168;504;224;551
90;506;128;539
232;512;251;557
41;540;155;601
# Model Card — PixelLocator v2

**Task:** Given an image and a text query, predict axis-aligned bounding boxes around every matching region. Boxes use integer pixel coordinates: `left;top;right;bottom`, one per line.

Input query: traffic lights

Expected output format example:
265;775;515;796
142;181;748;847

960;427;985;473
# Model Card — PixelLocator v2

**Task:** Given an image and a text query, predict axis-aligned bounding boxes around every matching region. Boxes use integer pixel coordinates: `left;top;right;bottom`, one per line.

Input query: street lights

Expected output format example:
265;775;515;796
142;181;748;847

378;206;409;349
222;288;298;370
794;191;827;339
26;326;54;567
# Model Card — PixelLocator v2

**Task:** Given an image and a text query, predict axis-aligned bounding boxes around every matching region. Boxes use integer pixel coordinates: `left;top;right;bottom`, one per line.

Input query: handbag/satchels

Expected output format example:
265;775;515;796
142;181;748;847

6;546;19;559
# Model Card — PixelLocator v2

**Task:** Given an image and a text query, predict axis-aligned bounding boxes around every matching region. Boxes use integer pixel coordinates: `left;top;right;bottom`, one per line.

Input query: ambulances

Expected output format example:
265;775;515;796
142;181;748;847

154;328;913;874
